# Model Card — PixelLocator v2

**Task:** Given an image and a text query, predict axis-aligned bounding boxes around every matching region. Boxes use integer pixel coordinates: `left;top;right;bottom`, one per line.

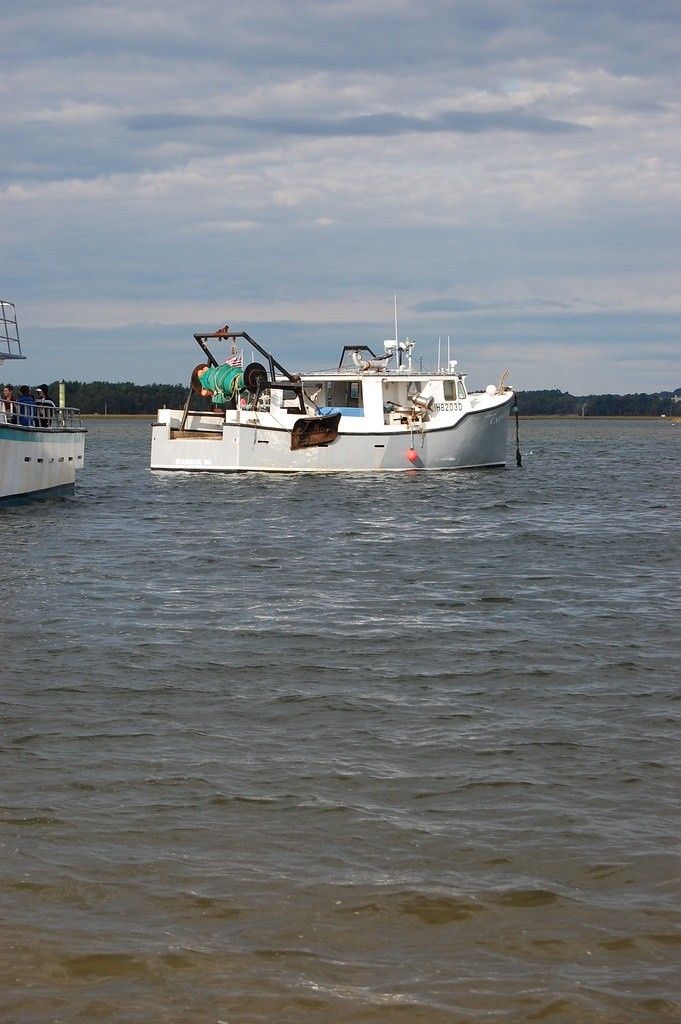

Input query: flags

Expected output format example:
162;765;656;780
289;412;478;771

223;352;242;369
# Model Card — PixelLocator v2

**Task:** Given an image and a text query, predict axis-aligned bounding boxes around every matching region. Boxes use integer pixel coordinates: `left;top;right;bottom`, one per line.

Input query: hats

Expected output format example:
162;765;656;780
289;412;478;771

36;388;42;392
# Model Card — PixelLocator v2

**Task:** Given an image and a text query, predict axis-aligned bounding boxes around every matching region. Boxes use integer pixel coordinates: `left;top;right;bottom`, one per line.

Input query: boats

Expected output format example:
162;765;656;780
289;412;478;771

150;291;519;472
0;300;89;505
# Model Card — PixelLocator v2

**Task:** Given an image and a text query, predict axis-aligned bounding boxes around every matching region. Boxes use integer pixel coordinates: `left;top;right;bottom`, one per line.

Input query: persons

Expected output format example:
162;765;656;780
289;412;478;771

240;392;259;411
3;384;60;427
